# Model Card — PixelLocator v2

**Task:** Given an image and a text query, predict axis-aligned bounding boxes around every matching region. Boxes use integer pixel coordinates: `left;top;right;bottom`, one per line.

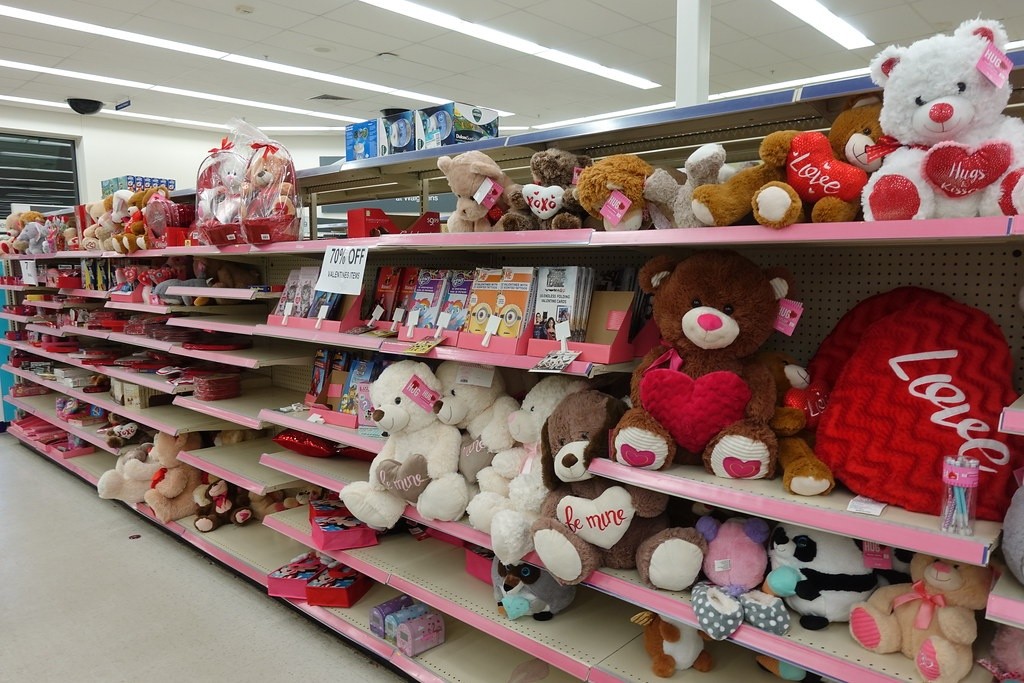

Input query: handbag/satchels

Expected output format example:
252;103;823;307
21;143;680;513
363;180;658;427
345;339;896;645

263;499;495;608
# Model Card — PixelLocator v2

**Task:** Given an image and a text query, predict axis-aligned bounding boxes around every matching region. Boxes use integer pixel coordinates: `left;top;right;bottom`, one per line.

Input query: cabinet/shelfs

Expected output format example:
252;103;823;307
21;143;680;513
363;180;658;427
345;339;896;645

164;105;645;683
586;48;1024;683
0;189;308;557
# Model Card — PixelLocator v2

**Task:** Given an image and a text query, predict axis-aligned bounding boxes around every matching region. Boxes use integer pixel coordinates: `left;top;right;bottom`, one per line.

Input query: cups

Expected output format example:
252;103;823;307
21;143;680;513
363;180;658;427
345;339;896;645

941;455;980;536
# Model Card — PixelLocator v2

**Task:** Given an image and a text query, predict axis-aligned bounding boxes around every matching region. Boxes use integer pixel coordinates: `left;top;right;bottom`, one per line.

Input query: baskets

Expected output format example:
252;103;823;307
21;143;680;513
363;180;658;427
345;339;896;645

194;151;248;247
242;142;300;244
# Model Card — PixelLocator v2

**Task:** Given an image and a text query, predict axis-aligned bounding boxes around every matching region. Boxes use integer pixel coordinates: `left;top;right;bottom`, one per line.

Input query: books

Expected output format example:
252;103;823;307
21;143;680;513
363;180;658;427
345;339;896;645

273;266;652;434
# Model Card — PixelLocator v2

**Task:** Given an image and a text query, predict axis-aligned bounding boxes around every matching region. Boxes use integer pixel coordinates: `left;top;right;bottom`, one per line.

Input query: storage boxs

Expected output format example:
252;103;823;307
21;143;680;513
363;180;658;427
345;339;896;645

455;315;534;355
422;103;498;146
345;119;381;161
527;290;636;364
396;327;462;346
379;107;419;156
269;283;368;333
100;176;175;198
630;316;663;356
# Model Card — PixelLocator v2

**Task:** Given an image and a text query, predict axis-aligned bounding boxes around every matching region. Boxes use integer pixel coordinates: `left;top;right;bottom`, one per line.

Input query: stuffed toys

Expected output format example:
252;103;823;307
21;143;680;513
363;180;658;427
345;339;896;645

340;19;1024;682
0;153;323;533
